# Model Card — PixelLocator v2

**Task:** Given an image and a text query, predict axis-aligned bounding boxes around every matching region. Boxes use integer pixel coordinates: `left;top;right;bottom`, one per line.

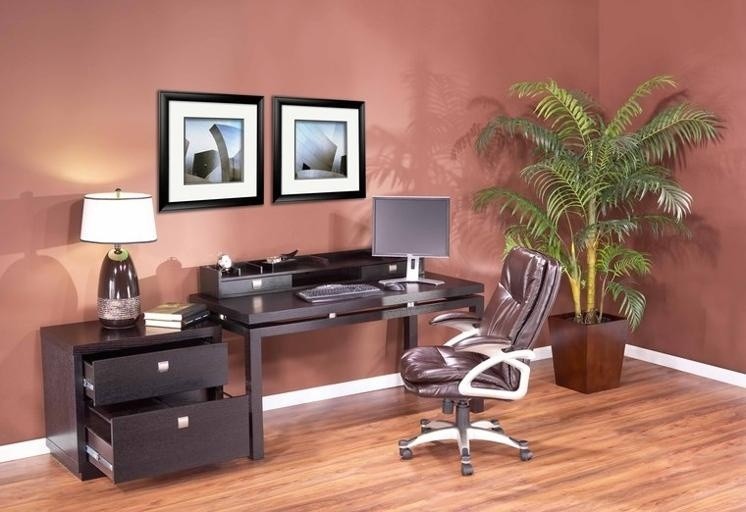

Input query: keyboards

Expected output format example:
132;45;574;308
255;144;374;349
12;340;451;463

296;283;383;303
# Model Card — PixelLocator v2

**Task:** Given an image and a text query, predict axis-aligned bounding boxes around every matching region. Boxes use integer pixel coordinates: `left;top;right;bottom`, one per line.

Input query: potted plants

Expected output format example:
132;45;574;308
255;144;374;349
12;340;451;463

469;56;731;394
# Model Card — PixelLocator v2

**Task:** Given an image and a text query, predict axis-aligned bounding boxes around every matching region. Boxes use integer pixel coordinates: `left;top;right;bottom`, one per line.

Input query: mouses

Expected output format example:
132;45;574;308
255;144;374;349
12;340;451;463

386;282;405;291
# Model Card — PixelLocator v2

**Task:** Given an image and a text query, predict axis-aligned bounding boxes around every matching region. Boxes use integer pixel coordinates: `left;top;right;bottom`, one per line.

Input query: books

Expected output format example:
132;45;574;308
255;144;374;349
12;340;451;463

145;327;181;335
143;302;210;330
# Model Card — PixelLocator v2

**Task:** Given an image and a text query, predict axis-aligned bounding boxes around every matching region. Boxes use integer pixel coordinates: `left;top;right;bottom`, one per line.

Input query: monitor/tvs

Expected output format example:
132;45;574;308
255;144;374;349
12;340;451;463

371;196;451;285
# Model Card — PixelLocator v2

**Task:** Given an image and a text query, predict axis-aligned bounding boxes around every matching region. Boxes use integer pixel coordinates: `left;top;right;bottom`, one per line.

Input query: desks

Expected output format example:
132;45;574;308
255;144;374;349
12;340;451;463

182;246;487;469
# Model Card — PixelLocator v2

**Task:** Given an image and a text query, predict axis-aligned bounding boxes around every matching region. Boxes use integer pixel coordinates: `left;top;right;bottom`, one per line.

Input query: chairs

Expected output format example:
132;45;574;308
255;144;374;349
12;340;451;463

395;239;567;481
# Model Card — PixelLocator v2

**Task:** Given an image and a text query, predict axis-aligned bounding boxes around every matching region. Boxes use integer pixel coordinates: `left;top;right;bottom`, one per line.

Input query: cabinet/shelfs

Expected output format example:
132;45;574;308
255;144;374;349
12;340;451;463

31;301;252;490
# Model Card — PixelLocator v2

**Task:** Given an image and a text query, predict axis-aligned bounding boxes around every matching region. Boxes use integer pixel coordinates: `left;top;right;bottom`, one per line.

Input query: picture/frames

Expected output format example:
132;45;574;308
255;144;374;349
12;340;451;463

266;91;369;208
151;87;268;216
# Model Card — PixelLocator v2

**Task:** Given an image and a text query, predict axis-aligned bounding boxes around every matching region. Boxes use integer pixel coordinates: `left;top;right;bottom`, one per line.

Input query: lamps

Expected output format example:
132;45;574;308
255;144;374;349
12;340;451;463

78;185;161;333
